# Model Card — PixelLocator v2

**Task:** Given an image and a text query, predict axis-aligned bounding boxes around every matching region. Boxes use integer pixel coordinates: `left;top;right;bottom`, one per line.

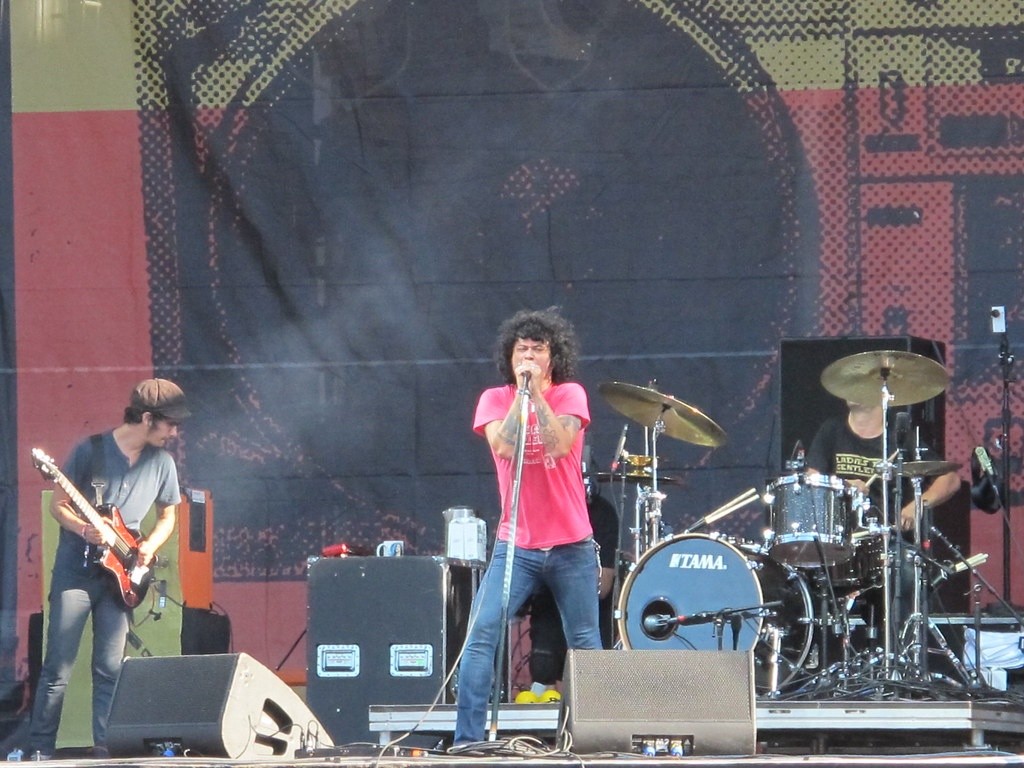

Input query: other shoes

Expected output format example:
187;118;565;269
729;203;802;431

30;752;51;760
87;747;109;759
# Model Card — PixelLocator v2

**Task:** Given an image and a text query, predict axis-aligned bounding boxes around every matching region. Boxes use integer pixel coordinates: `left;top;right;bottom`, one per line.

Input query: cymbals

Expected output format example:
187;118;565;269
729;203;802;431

598;381;729;448
819;348;951;407
597;454;677;485
892;460;963;479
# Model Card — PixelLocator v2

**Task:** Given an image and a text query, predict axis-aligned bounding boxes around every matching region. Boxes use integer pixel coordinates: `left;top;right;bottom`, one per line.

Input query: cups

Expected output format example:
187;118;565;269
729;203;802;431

377;540;404;557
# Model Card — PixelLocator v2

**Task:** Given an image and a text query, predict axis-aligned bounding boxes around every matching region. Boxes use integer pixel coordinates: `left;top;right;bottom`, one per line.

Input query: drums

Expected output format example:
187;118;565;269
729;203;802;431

616;531;816;696
767;471;855;570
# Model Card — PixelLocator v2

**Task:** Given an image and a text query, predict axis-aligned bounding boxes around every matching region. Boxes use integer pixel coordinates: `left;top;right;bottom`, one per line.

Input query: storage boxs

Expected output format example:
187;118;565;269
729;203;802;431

304;556;507;754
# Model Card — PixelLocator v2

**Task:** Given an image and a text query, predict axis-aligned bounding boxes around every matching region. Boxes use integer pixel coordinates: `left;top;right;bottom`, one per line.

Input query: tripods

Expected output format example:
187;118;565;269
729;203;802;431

767;369;975;701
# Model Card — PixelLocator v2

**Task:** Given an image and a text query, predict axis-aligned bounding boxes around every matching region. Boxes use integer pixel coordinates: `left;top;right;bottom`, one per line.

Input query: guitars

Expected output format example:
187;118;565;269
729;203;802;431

30;445;156;611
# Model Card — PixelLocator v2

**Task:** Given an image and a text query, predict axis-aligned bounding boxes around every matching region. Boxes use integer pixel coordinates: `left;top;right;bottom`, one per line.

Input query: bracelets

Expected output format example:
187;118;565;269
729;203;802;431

82;524;93;539
517;389;523;395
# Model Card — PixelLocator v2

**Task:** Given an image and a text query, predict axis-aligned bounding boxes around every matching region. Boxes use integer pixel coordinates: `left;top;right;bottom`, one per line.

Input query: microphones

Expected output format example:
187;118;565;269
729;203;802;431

611;424;628;473
797;439;809;474
644;613;669;635
922;498;931;557
521;371;531;380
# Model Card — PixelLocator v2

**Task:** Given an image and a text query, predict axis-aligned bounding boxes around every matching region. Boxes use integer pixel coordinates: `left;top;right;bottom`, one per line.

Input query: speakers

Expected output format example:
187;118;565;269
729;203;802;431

104;652;335;758
555;648;756;755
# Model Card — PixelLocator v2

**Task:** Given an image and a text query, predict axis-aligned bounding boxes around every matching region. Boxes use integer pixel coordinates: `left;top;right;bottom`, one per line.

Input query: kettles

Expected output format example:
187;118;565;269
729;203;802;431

443;505;478;557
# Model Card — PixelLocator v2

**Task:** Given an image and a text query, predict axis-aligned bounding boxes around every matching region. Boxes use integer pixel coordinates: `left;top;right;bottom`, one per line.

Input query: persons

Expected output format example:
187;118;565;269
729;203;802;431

3;379;191;757
804;400;962;699
453;312;603;743
514;493;624;697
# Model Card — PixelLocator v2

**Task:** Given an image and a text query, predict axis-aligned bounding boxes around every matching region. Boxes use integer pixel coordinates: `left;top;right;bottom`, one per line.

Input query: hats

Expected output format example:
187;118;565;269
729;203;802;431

132;378;192;418
